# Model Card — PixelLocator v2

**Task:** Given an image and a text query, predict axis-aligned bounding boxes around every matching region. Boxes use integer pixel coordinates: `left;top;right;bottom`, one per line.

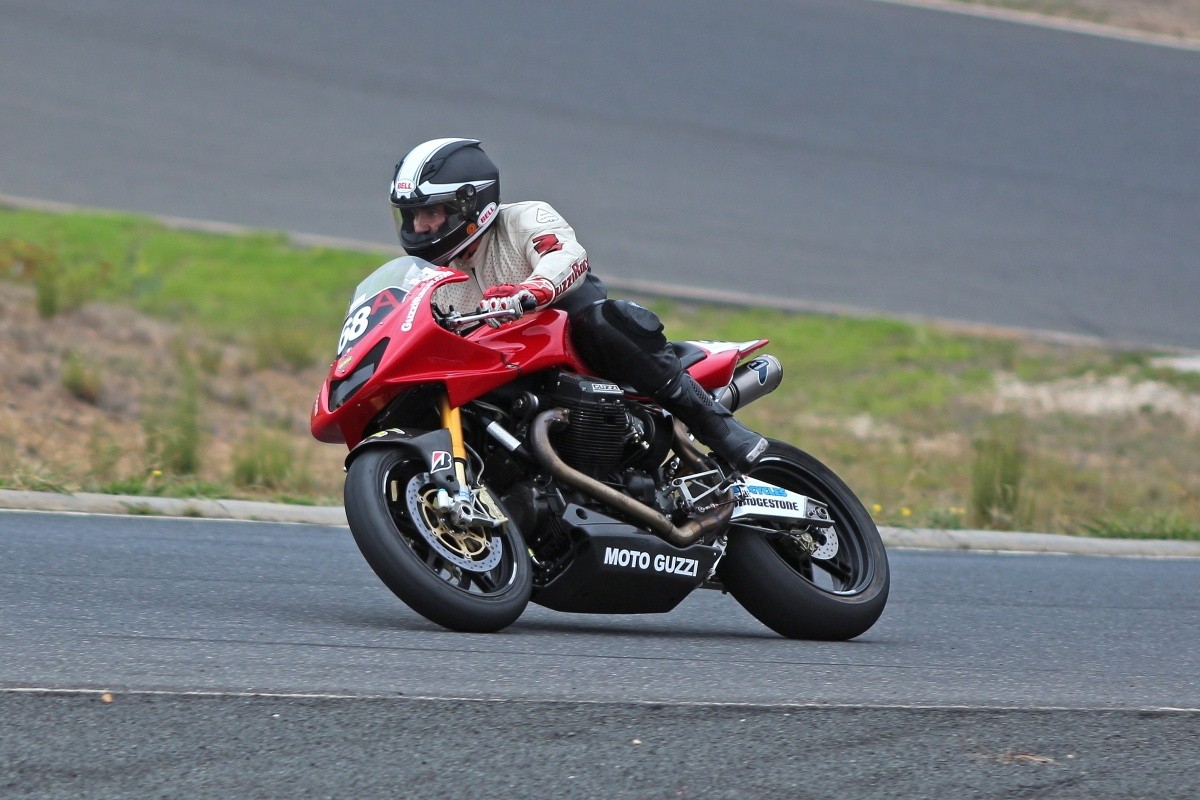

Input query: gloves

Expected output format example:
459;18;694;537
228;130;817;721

480;279;554;328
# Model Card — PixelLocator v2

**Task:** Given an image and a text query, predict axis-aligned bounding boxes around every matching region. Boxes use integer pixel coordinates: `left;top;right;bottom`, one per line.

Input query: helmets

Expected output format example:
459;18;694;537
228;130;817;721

389;138;500;270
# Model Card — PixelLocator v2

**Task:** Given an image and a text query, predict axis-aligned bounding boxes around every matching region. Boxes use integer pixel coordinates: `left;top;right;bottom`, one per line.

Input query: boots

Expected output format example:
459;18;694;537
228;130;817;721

656;371;767;466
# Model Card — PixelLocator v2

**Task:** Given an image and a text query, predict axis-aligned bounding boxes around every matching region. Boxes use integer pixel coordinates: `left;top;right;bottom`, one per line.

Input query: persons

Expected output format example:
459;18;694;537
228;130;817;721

385;136;769;474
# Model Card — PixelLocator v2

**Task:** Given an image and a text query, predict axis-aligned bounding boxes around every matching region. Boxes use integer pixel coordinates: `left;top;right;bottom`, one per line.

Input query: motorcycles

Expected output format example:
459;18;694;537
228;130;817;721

310;256;891;643
